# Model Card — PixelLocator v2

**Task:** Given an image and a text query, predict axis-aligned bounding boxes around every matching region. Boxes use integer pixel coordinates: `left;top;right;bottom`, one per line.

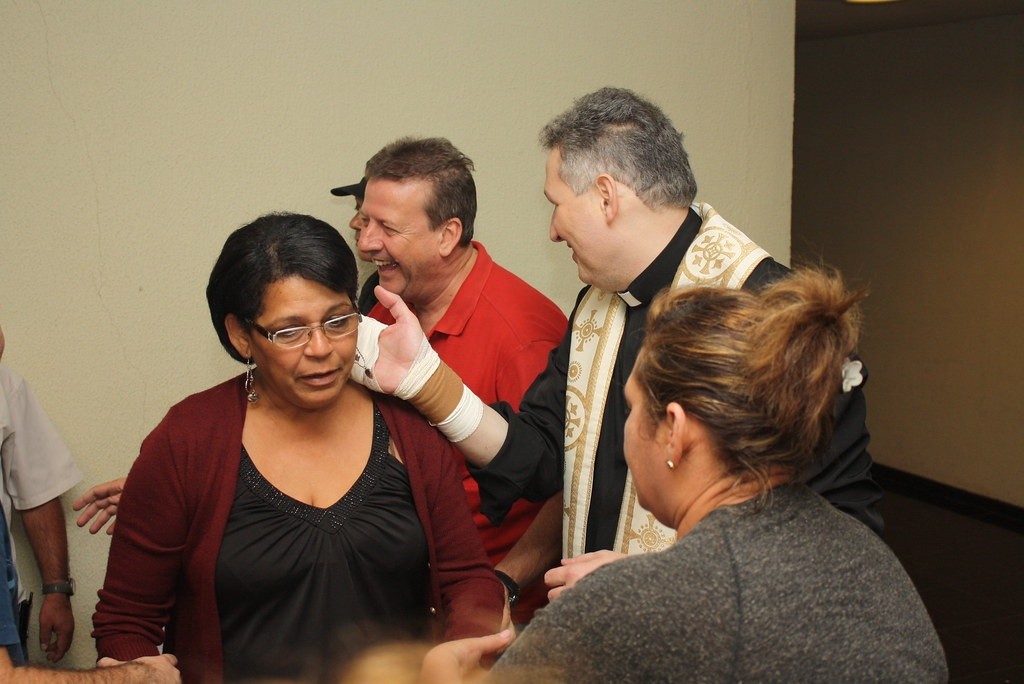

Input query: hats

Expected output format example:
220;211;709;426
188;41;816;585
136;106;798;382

329;176;367;202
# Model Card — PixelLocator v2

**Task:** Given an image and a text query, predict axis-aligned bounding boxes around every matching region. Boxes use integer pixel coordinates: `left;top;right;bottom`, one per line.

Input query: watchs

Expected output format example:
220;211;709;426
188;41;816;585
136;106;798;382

42;579;76;596
495;569;521;606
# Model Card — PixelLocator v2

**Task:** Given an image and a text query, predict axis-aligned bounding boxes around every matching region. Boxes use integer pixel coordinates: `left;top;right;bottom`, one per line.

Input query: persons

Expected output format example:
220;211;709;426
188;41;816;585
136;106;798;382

0;330;182;684
91;213;504;684
330;177;380;315
346;86;885;602
73;135;569;637
421;269;948;684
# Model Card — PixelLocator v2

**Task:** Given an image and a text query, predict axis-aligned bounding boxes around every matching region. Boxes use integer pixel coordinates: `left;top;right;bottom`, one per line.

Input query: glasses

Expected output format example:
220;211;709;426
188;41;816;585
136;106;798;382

246;299;363;349
